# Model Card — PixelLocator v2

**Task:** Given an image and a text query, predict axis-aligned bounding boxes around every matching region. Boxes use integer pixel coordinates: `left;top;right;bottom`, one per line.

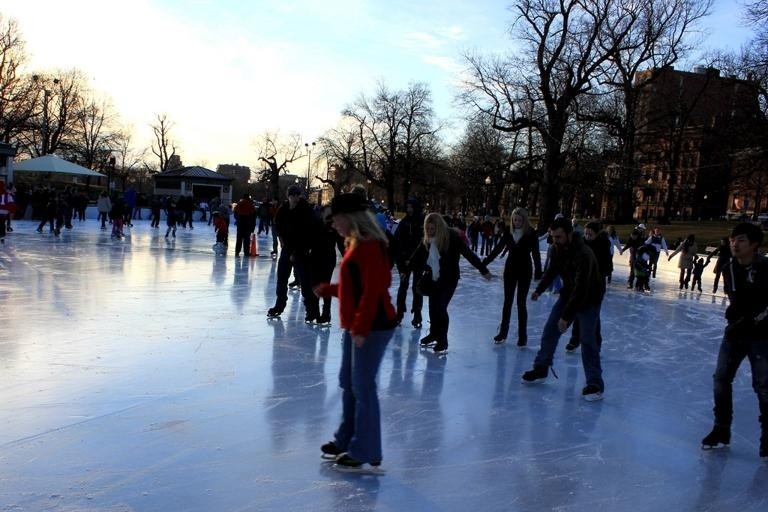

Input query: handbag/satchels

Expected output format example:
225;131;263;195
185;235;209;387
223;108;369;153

415;270;432;297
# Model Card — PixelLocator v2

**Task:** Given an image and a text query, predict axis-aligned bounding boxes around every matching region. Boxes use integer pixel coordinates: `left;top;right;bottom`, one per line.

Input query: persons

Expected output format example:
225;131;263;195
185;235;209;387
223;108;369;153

1;178;768;467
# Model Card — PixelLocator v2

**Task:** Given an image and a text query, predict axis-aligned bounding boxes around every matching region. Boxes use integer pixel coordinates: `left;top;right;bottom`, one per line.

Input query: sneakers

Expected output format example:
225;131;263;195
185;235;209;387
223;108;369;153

335;453;384;468
581;382;605;396
420;328;438;345
433;338;448;352
566;343;577;351
759;433;768;457
321;437;349;455
701;424;730;446
522;357;548;382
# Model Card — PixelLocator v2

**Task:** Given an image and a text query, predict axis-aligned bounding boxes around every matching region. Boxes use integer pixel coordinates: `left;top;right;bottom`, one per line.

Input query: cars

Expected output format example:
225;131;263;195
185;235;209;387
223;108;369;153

714;209;767;232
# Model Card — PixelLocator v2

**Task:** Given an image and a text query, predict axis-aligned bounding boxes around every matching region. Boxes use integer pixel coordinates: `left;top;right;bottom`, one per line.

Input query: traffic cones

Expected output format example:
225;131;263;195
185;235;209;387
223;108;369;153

250;234;260;257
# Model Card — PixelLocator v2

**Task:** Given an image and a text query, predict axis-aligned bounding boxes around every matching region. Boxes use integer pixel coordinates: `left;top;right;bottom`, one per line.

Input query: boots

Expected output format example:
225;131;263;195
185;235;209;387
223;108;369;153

411;310;422;326
516;328;528;347
268;297;288;316
494;325;507;341
316;304;331;323
396;310;405;323
305;309;320;321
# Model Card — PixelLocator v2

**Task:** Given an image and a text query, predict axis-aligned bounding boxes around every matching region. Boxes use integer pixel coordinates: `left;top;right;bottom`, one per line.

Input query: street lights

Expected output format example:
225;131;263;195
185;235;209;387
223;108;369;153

305;140;316;190
344;133;355;192
480;172;494;220
645;176;657;227
31;74;59;156
108;154;117;196
367;177;374;201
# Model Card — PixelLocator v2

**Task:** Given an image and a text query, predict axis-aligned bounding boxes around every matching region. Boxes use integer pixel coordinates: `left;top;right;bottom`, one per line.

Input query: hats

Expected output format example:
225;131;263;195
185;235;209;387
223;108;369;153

324;192;370;223
637;223;648;230
285;185;302;197
